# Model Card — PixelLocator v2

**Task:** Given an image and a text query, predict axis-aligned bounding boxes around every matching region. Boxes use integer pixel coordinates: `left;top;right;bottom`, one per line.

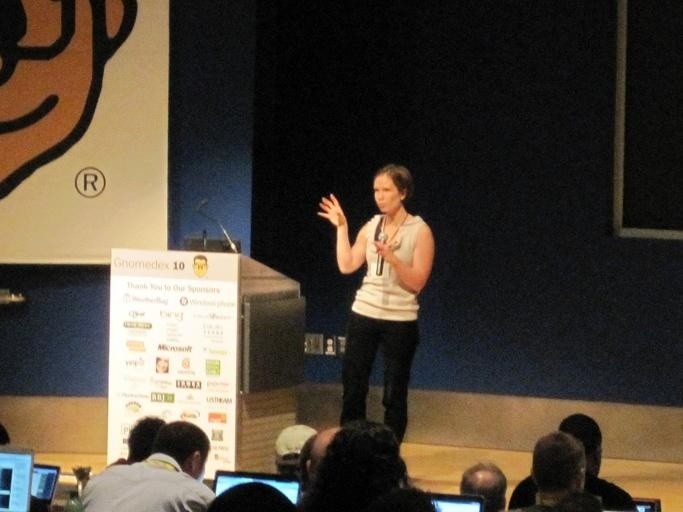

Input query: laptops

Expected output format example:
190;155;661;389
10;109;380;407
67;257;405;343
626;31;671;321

425;491;483;512
631;496;660;512
31;463;60;505
212;470;301;509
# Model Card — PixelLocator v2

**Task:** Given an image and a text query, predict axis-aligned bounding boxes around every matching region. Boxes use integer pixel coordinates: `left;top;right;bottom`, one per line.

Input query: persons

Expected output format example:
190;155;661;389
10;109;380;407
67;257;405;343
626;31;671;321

316;162;434;445
531;433;603;510
156;358;168;373
460;462;507;511
273;425;316;472
509;413;638;511
81;421;216;511
104;416;166;468
298;421;437;511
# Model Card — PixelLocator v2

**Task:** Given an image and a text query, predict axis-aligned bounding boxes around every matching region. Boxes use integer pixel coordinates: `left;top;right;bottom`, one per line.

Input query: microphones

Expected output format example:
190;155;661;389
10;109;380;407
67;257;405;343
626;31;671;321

196;198;238;253
376;232;387;276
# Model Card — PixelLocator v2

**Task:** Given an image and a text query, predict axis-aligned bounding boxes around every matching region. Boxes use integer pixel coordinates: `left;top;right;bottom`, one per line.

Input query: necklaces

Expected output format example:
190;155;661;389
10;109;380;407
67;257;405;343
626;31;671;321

380;212;408;244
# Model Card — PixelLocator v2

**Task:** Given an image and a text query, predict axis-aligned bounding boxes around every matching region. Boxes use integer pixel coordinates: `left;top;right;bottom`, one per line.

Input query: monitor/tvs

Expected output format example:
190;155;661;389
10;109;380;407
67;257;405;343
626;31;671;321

0;448;35;512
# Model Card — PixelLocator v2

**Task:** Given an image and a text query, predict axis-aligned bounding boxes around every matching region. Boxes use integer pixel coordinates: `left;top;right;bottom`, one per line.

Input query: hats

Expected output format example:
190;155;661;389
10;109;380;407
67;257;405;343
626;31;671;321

274;424;318;465
